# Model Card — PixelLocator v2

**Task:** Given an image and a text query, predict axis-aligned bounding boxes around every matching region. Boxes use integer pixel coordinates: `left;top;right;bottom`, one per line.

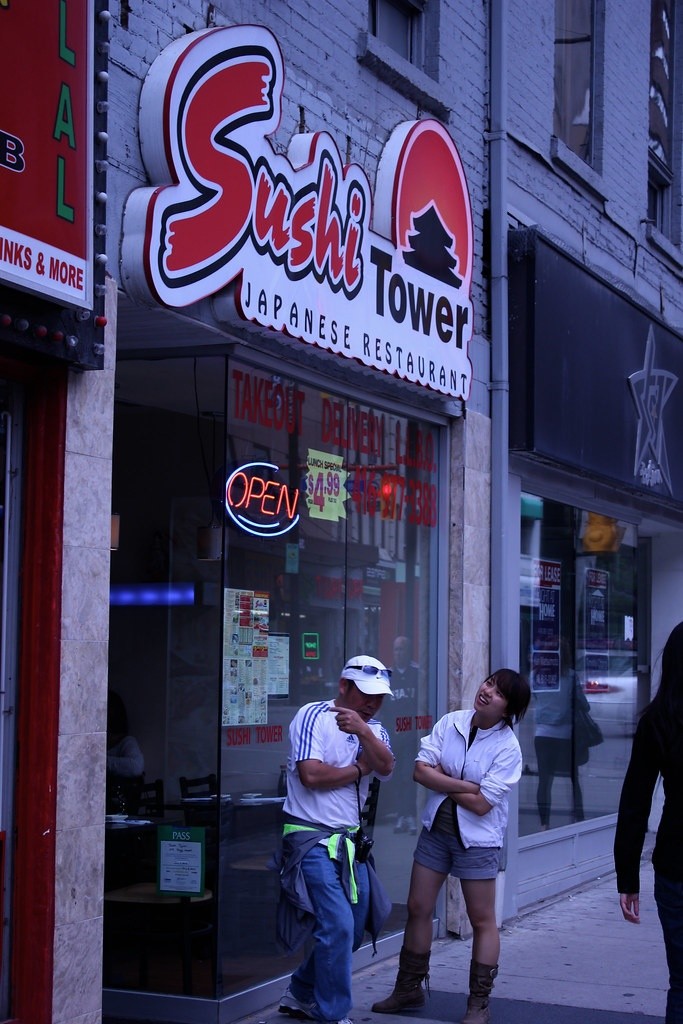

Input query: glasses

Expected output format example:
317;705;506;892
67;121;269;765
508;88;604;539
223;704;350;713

345;665;392;678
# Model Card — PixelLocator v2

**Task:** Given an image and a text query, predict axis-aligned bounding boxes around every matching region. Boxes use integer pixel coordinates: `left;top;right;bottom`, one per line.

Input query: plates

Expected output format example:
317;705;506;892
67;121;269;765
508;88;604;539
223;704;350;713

243;792;262;799
210;794;231;798
105;814;129;821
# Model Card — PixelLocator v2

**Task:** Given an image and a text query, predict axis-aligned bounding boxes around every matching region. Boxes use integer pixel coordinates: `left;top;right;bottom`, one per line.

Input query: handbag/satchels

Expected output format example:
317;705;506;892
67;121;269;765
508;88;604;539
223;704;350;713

576;705;604;748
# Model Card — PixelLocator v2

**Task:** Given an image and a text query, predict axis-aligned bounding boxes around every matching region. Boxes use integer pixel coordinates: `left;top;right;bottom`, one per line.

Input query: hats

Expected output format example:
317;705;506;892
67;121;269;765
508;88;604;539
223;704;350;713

340;654;395;697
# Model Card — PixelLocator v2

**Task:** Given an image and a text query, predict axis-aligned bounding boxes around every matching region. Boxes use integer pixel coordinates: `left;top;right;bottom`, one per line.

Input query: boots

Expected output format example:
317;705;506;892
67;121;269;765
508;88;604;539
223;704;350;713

462;959;499;1024
371;945;432;1013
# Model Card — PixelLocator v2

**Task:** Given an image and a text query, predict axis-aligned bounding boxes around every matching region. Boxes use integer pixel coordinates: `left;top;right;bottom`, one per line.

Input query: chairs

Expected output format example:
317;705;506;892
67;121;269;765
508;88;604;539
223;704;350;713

104;760;381;993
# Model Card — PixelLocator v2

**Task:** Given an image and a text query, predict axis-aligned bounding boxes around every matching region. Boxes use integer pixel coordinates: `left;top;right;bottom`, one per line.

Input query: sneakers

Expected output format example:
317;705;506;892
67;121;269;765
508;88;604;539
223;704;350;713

277;988;317;1020
336;1017;354;1024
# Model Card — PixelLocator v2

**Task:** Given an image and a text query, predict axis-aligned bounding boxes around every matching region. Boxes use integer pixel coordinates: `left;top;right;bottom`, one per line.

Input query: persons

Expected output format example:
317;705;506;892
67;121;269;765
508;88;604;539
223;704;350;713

530;634;603;833
105;691;146;776
383;636;419;835
277;652;393;1024
370;668;531;1024
611;622;683;1024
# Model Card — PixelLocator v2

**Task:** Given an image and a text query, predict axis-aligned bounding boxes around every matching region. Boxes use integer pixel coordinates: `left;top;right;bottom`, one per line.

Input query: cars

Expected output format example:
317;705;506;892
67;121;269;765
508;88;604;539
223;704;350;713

578;653;637;745
